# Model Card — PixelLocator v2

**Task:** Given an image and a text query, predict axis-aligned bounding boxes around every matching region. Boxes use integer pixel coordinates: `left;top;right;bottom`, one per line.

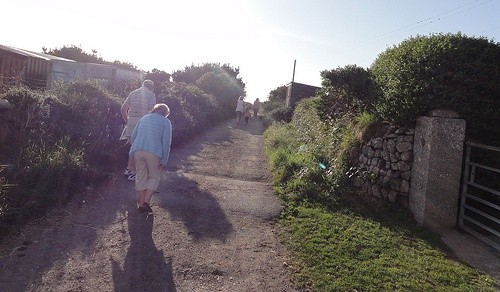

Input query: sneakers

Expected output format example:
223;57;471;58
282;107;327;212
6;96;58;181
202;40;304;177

124;169;131;175
127;172;135;181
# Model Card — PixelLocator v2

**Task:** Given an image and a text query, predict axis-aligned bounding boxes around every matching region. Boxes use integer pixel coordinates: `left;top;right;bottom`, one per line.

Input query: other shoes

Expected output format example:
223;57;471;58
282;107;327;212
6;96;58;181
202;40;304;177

142;202;152;212
137;204;145;212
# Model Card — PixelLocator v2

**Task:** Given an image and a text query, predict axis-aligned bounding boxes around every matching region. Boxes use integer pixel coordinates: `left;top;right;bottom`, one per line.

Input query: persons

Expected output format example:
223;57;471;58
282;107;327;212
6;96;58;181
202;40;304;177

128;103;172;213
253;98;260;118
235;96;244;125
244;106;251;128
122;80;157;180
